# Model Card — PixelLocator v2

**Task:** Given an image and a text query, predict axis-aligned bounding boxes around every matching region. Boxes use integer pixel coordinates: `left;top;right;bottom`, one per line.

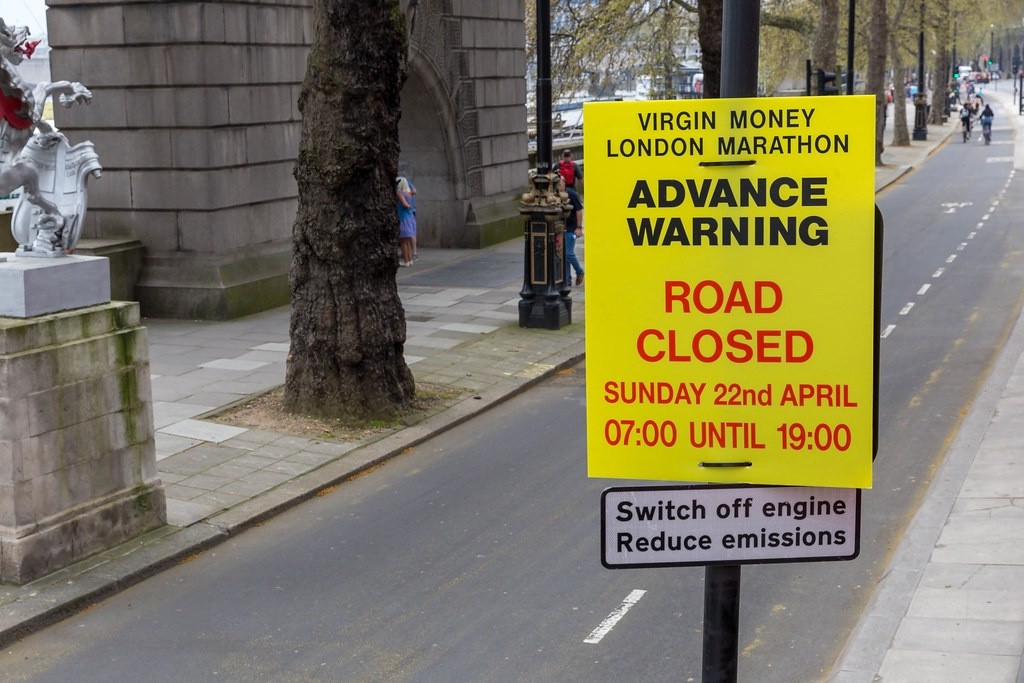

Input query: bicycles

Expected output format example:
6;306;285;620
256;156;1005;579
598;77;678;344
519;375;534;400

980;117;992;144
961;117;972;143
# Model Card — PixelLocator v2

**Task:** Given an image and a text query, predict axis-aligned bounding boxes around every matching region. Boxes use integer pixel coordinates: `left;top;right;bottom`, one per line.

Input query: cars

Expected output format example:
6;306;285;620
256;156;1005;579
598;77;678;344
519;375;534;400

969;72;990;83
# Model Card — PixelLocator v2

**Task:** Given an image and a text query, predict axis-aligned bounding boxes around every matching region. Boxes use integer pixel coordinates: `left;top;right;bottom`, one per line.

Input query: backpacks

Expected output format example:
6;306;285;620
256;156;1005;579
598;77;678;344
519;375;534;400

559;160;575;185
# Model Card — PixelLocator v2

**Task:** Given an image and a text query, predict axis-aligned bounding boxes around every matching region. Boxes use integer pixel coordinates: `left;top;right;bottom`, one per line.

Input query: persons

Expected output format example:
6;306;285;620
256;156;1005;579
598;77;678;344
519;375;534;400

959;104;972;137
906;83;911;98
395;175;419;266
557;175;584;286
946;78;962;108
966;82;974;98
979;104;994;140
553;149;582;192
983;75;989;84
978;87;984;104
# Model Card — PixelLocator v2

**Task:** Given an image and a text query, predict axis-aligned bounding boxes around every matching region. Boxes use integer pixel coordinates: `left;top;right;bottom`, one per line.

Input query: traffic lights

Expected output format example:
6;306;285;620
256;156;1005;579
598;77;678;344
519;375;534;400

816;68;839;96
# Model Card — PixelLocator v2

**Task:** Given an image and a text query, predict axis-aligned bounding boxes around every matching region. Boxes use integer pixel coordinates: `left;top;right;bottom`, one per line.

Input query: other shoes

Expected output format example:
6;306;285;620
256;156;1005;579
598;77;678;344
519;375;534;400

399;260;414;267
575;273;584;286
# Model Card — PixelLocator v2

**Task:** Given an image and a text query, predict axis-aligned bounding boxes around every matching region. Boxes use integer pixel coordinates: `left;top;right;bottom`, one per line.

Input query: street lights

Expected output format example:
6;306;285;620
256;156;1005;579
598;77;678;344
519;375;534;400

990;23;994;81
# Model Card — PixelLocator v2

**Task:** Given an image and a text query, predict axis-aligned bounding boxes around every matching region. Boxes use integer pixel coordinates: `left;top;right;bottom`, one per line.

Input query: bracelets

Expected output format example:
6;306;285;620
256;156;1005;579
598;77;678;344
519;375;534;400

577;226;582;228
